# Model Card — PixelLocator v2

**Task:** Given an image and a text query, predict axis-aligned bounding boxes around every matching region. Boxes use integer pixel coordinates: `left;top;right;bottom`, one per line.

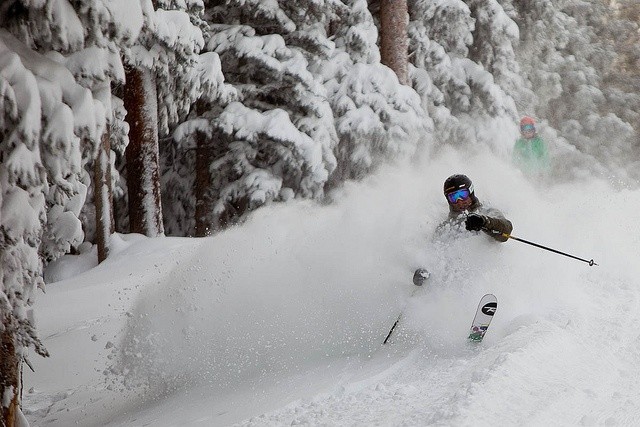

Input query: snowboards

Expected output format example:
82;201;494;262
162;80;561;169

468;293;498;346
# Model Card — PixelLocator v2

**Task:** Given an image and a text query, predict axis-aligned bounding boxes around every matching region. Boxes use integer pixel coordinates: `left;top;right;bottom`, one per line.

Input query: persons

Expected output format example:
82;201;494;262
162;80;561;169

412;174;514;287
512;115;551;188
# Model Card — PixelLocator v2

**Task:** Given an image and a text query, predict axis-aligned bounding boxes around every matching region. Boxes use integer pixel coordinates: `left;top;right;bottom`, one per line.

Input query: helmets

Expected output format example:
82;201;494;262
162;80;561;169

444;174;480;212
520;116;535;131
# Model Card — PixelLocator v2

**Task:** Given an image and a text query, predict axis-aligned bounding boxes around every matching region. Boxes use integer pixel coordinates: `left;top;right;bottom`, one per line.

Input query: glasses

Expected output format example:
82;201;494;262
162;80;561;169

521;124;534;129
446;188;470;203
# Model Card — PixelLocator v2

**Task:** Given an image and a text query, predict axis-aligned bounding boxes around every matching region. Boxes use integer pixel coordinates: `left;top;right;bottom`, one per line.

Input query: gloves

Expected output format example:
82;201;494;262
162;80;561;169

413;268;430;286
465;213;485;231
